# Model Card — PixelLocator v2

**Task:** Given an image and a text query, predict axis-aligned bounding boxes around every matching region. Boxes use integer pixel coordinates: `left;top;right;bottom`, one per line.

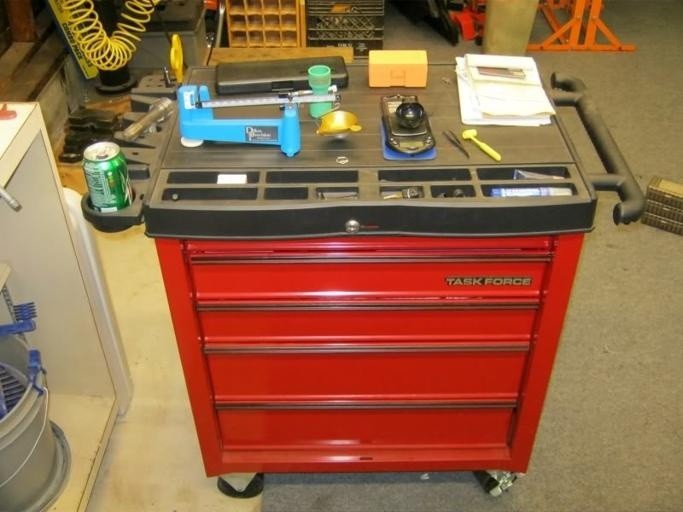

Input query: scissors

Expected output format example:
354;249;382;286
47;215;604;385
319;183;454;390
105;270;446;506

170;33;185;87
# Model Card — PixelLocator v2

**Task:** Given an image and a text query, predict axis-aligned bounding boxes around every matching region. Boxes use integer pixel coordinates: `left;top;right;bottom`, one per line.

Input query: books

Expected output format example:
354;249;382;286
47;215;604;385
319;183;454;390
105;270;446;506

454;52;556;129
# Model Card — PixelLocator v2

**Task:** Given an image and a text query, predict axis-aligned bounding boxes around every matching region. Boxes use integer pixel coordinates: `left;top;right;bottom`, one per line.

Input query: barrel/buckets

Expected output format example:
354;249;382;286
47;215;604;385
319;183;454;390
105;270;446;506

0;333;53;512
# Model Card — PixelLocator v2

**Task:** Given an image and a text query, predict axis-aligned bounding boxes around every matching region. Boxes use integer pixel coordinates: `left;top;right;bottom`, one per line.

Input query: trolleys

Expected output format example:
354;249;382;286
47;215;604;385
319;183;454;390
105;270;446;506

82;63;647;497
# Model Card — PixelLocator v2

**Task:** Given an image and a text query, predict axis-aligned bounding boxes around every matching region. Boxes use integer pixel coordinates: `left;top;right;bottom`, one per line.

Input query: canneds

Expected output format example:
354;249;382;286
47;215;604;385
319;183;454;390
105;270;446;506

81;141;133;214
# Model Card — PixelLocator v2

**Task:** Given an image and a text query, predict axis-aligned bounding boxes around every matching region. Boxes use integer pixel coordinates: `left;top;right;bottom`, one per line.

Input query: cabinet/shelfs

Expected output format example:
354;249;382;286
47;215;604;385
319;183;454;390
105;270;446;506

0;100;135;512
